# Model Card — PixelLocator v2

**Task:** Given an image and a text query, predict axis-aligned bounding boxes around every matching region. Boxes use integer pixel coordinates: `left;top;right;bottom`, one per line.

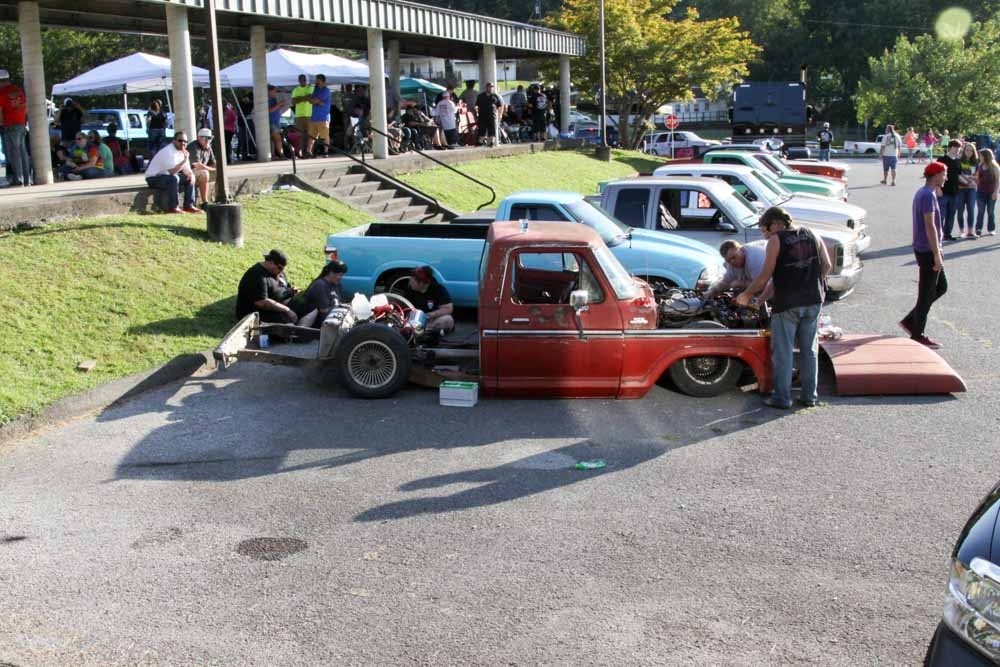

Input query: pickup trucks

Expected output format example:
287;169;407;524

844;135;928;157
324;192;726;312
211;219;774;402
51;108;175;140
450;144;866;308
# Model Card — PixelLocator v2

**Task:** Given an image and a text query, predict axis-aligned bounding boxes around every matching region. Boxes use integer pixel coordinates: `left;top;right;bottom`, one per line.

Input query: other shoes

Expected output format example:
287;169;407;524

942;228;996;242
271;151;328;160
68;174;82;180
388;135;547;156
795;398;815;407
201;202;208;210
183;206;200;212
169;207;183;213
763;398;794;409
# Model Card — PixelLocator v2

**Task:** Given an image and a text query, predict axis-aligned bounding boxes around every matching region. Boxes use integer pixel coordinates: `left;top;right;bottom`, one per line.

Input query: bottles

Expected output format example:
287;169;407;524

819;313;830;328
574;459;606;469
260;333;268;350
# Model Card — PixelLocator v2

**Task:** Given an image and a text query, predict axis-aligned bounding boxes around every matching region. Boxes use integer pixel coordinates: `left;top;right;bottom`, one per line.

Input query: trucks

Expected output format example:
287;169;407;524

728;81;814;151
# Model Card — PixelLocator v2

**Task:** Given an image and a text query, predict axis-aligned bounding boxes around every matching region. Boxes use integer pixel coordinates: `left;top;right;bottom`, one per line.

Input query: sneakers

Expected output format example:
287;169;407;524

898;318;915;335
911;335;943;349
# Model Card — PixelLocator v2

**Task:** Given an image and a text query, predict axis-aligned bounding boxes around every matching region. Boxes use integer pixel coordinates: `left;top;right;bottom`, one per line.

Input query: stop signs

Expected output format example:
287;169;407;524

664;115;679;129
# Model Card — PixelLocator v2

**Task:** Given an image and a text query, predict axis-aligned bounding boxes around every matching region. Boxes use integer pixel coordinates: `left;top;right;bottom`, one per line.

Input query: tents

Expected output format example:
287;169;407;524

47;51;257;148
400;76;447;117
202;48;388;148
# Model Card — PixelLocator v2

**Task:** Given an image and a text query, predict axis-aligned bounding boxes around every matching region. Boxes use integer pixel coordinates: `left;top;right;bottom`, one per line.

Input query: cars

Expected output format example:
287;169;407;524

639;131;732;157
567;109;602;140
922;477;1000;667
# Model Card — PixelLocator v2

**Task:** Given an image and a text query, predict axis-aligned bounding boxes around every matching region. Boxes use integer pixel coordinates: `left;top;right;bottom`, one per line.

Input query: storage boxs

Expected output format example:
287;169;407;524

439;381;478;407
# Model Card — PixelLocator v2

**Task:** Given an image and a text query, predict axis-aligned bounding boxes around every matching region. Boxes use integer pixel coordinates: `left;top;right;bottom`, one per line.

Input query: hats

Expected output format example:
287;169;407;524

0;70;12;78
198;128;212;137
264;249;288;266
920;162;949;178
824;122;830;128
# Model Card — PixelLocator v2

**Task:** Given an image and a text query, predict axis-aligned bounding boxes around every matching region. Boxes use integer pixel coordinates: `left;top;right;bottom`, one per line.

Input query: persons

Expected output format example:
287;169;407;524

898;161;948;348
145;128;216;214
510;82;560;141
880;125;902;186
816;121;834;161
54;97;168;189
236;249;347;328
701;206;831;408
903;127;954;164
195;88;254;165
267;74;504;156
935;139;1000;241
0;69;29;188
396;266;456;334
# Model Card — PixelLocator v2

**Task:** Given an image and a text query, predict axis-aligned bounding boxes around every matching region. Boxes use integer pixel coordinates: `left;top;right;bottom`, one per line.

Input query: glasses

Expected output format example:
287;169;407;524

199;136;212;139
177;139;188;143
76;136;86;141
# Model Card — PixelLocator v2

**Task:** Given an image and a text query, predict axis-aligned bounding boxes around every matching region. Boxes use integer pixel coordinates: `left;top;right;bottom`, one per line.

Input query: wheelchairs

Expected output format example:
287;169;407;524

348;129;427;153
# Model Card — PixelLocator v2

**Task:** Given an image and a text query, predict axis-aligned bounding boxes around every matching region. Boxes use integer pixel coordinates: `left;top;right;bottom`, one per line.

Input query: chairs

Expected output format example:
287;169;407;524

657;205;679;230
350;117;370;154
